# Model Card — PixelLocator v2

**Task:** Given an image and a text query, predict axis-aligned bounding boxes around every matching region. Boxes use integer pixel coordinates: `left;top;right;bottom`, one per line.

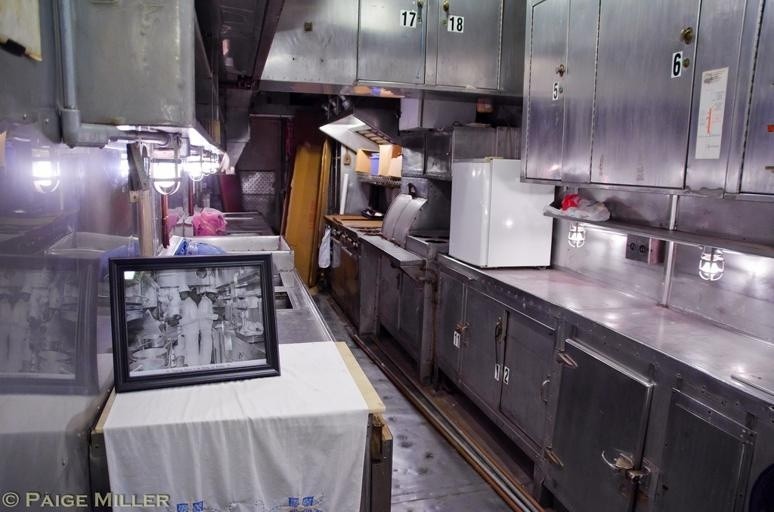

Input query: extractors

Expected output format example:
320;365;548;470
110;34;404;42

355;128;396;145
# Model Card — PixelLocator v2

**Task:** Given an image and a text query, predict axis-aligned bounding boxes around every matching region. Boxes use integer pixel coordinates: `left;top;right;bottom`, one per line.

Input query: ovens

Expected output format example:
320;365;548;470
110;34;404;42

321;222;360;336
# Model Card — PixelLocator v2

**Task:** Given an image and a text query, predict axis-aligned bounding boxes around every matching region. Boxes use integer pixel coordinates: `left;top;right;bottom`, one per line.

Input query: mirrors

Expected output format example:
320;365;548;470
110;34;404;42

109;253;280;393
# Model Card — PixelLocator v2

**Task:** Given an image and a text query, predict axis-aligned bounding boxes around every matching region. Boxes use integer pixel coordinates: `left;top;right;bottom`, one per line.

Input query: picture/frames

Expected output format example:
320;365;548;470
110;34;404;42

0;255;101;395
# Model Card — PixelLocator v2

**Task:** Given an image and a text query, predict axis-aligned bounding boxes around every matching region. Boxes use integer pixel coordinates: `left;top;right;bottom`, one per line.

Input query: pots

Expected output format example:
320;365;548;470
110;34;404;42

36;349;70;375
131;347;168;369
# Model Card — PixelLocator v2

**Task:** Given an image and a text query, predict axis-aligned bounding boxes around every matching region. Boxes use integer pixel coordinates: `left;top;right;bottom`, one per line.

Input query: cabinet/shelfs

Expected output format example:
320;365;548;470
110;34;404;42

258;0;525;99
521;0;774;202
53;0;228;154
328;223;360;325
376;238;425;361
430;267;774;512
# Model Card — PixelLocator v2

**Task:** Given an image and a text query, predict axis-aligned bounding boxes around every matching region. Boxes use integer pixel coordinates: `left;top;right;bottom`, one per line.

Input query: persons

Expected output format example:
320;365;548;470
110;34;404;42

197;286;220;367
176;285;198;367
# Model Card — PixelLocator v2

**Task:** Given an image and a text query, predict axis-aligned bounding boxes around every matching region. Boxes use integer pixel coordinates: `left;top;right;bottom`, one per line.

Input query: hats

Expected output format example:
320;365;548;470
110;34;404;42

205;286;218;293
179;282;191;292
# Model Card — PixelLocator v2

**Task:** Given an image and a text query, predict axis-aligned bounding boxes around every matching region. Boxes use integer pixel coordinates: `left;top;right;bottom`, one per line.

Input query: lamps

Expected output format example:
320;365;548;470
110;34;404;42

149;133;220;196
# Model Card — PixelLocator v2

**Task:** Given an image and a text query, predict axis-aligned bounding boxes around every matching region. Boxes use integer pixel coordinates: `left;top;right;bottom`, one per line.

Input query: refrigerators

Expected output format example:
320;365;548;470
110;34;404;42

446;158;554;271
